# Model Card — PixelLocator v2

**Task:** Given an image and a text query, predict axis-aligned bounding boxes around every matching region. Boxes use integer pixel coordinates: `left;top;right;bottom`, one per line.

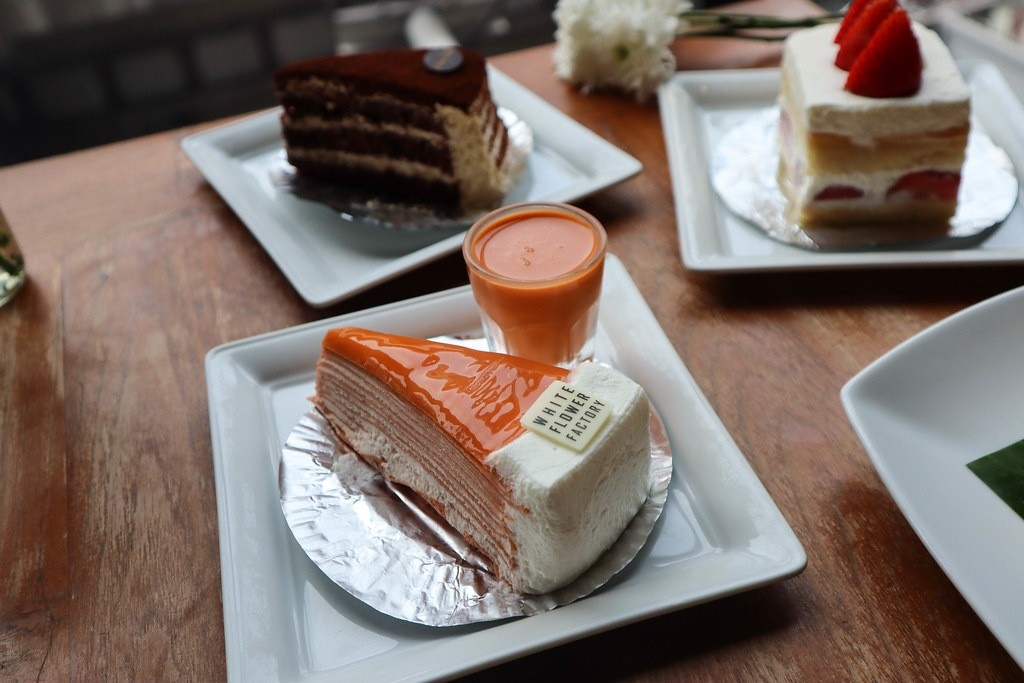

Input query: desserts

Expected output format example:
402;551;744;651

272;45;508;216
312;326;651;593
774;0;970;229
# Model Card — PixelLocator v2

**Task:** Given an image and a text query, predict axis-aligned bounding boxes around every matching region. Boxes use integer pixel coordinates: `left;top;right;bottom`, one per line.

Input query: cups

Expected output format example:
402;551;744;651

463;201;614;370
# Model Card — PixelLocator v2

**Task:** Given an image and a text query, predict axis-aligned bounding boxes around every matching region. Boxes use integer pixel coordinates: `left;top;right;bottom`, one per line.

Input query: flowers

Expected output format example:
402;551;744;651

551;0;847;100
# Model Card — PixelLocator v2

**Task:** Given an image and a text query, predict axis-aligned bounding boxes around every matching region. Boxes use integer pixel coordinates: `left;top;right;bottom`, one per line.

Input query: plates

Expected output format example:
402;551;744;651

653;64;1022;271
204;251;808;677
838;285;1022;678
180;53;645;308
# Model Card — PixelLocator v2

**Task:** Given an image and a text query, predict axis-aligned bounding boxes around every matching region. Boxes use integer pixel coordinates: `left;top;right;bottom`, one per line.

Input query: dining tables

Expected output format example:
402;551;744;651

0;0;1023;681
